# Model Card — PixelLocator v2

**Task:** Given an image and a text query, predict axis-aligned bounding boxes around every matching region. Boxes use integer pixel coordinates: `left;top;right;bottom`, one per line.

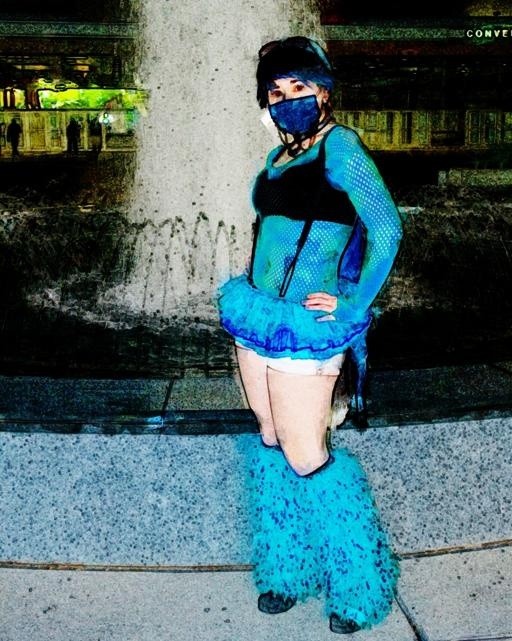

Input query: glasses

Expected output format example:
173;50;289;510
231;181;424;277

259;38;329;68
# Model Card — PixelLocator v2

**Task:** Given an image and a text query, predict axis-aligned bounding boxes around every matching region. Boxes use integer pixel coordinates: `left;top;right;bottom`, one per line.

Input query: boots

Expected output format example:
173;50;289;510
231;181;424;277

296;452;398;634
258;434;317;613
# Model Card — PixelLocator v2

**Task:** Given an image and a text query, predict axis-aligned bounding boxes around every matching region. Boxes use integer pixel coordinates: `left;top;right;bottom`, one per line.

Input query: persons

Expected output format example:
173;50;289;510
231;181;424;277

217;32;404;631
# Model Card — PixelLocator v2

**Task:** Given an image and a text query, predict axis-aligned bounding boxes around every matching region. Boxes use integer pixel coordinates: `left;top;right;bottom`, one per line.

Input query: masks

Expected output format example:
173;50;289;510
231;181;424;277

268;95;321;136
